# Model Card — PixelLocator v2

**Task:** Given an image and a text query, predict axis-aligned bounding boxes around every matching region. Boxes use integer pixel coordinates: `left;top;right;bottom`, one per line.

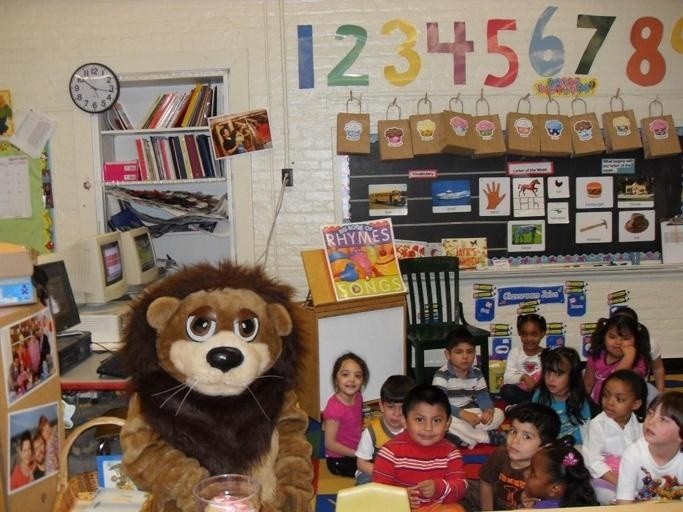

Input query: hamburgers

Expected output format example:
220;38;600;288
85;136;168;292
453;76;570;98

586;183;602;198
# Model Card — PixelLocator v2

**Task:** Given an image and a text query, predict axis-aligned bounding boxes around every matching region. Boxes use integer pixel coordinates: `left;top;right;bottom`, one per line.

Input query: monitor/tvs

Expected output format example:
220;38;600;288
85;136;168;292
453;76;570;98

32;260;81;333
120;226;159;291
37;231;128;314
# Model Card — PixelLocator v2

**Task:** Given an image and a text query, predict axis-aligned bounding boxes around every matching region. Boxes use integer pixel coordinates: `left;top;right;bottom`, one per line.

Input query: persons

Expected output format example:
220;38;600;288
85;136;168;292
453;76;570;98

613;306;666;391
570;369;649;506
522;441;604;509
318;354;369;476
532;345;592;448
500;313;549;401
431;326;509;448
10;431;35;489
348;373;417;487
369;385;470;512
581;312;650;407
31;431;47;478
483;181;506;209
215;115;269;155
37;413;60;475
3;315;51;395
478;401;562;512
614;390;683;503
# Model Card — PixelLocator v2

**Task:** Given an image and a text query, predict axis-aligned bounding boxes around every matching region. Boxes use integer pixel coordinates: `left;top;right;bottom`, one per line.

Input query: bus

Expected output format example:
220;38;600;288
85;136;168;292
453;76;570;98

371;189;406;208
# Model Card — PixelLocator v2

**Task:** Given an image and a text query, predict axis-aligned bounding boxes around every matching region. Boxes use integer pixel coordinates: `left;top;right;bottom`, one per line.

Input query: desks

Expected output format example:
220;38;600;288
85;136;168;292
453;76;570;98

58;351;136;391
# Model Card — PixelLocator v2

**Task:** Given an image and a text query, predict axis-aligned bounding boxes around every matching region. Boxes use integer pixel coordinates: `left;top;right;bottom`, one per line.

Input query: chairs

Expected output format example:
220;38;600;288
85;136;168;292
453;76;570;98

398;255;491;395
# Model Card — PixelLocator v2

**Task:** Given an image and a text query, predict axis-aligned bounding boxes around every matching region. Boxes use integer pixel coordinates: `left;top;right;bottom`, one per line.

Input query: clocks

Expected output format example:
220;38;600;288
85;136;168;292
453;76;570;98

68;62;120;114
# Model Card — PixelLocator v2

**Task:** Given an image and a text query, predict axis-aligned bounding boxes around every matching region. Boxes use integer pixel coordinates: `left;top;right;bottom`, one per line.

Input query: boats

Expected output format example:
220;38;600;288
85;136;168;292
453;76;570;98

435;189;467;200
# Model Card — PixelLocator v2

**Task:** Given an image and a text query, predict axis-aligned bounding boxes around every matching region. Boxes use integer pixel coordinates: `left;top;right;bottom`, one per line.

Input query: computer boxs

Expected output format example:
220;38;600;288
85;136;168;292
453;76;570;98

58;300;137;343
54;328;92;377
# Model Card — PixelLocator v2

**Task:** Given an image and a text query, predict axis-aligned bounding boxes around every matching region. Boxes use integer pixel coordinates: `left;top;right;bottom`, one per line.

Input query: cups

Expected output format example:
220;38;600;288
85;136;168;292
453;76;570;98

192;474;263;512
630;251;639;265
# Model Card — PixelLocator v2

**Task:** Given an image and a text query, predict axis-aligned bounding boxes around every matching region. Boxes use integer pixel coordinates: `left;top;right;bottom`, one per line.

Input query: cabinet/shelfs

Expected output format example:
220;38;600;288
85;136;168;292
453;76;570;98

88;69;235;298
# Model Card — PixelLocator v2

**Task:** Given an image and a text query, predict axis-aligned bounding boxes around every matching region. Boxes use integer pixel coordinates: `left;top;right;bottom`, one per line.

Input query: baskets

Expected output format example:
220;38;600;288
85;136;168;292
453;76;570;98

52;416;157;512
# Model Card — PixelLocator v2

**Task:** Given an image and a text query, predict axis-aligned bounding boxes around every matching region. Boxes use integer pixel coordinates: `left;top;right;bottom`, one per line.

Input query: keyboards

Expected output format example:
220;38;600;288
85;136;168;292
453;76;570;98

96;352;130;379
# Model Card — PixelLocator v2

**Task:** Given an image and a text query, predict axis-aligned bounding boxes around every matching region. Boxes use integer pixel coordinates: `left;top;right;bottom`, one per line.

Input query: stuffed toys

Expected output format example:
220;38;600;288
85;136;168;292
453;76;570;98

118;257;315;511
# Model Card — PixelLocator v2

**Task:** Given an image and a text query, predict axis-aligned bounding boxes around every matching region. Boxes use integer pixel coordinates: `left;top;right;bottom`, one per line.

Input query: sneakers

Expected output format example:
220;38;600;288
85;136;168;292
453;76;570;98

488;429;509;445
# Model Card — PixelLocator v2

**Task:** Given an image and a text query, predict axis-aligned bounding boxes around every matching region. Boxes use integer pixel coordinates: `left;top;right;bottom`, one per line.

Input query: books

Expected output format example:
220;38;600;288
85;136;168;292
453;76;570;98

320;218;409;302
102;82;227;181
